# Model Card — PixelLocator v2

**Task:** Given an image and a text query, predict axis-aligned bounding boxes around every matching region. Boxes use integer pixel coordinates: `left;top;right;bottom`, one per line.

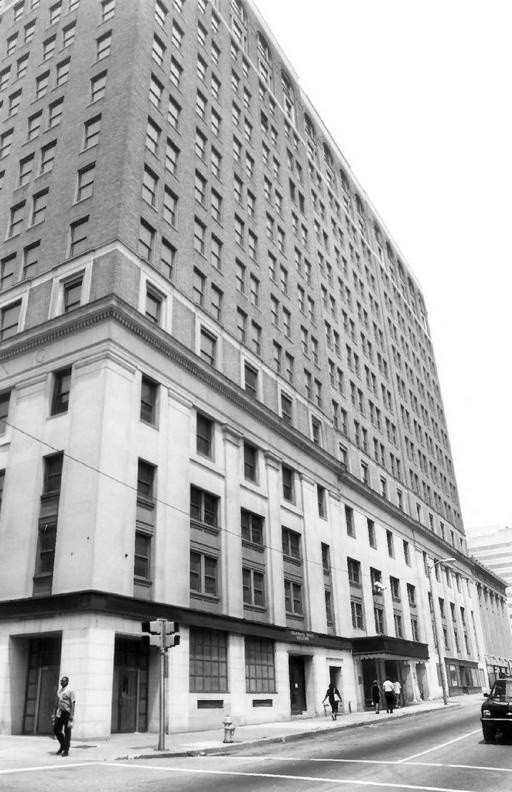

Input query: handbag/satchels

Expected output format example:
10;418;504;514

334;694;341;701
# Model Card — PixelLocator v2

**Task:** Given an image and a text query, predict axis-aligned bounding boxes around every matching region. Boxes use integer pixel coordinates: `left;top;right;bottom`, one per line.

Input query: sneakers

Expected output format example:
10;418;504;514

332;713;337;719
56;749;67;756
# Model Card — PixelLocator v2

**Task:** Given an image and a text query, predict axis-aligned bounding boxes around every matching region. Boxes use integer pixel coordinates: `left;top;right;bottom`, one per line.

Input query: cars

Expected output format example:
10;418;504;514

480;676;512;742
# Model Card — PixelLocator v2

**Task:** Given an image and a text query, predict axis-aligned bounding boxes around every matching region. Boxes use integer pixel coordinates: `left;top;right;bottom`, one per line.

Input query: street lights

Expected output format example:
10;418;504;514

427;558;457;704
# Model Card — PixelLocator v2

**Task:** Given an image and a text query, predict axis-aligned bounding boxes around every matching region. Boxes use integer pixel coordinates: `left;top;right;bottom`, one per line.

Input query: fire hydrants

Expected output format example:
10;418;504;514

222;714;236;743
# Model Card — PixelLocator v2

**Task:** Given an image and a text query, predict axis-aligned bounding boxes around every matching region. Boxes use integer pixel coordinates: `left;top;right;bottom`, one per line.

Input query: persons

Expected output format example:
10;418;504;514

393;677;402;708
321;680;342;720
51;675;76;755
371;679;383;713
382;676;396;713
